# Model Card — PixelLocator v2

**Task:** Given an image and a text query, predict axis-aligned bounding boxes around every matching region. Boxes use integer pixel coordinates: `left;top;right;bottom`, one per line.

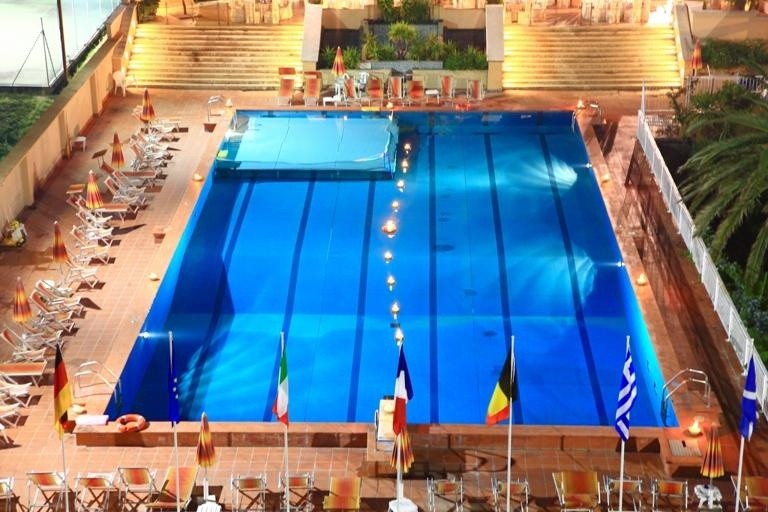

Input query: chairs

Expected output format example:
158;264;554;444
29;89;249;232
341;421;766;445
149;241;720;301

117;465;159;511
275;66;489;112
73;471;117;512
51;184;139;289
112;71;139;98
0;474;21;511
491;477;529;512
516;1;650;26
26;469;72;512
0;362;47;449
146;464;200;511
427;472;461;512
92;105;180;208
278;471;314;512
552;471;600;512
0;279;83;361
650;478;688;512
322;477;361;512
730;475;768;512
602;473;643;512
222;1;296;26
231;471;267;512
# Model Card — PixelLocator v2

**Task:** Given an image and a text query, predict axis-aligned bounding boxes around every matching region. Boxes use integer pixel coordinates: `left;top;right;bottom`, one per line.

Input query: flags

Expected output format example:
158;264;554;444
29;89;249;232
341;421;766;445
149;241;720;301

485;349;519;425
271;344;289;425
392;343;414;435
53;342;74;440
740;348;760;443
614;343;639;442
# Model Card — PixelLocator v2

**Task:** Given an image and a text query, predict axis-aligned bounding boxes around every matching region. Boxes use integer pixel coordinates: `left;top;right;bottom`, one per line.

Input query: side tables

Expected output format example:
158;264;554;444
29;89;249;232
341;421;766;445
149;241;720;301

691;484;723;512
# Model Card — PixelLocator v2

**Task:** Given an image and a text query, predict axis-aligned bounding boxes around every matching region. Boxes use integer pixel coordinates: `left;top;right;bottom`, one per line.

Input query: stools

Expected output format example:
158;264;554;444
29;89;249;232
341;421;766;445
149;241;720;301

70;136;86;152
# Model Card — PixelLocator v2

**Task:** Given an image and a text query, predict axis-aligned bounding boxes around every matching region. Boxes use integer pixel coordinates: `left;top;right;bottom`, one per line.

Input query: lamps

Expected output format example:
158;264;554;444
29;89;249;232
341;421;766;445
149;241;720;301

379;138;415;353
585;162;592;169
637;272;647;286
616;261;626;267
600;171;609;183
576;96;584;109
689;416;703;436
194;173;203;182
226;97;233;108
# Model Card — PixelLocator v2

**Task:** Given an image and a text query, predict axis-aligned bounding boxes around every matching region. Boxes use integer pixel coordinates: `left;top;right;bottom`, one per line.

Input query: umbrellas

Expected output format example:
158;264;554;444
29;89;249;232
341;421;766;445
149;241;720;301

86;170;104;227
12;276;33;350
141;88;157;136
52;220;69;285
390;429;415;503
332;46;346;95
112;132;125;174
196;413;217;502
691;40;702;77
700;422;724;509
